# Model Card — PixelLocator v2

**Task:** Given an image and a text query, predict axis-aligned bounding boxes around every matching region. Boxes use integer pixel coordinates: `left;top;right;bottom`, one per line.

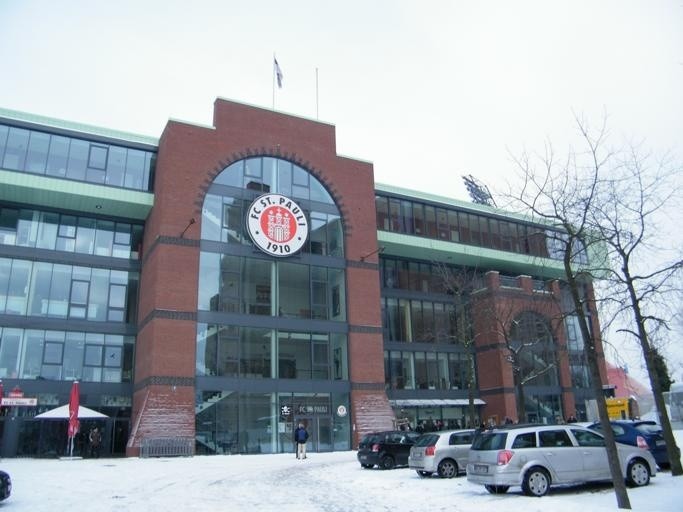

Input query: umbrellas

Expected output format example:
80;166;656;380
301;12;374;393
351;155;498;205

67;380;80;458
33;402;109;456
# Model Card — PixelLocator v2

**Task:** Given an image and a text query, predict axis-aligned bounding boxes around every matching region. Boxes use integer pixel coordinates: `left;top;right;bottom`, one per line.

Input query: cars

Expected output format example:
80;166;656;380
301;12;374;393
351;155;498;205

358;430;422;469
563;419;680;469
408;428;488;478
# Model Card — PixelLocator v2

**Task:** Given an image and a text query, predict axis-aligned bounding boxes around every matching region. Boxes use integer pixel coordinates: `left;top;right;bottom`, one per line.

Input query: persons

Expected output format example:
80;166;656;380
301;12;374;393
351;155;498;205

396;415;514;432
88;426;102;458
555;415;565;425
567;415;577;423
295;423;309;459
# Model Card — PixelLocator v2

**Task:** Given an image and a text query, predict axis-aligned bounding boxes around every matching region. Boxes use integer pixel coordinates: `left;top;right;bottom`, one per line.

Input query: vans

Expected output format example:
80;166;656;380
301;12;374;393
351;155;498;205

466;423;658;497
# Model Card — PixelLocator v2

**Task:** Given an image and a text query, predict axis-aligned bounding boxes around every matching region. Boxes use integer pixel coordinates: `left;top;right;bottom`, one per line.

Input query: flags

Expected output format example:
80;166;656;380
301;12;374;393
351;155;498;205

274;58;283;88
